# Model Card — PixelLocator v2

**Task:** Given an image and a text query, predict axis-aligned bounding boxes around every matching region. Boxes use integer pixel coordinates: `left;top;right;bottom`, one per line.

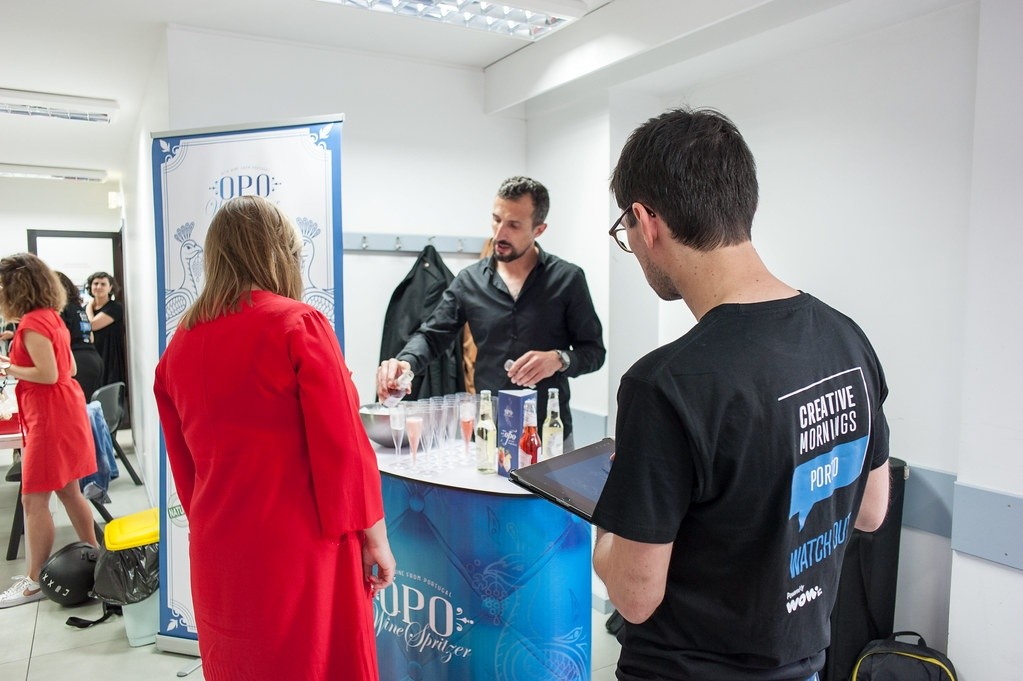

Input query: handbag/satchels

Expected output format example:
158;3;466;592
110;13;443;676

848;631;958;681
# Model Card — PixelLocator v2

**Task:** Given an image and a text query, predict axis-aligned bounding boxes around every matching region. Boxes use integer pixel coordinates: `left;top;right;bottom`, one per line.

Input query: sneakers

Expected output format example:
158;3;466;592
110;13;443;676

0;576;47;608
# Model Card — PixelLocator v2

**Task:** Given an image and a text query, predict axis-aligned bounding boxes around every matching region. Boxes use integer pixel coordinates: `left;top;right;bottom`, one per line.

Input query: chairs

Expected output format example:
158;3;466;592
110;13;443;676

5;400;114;560
92;382;143;487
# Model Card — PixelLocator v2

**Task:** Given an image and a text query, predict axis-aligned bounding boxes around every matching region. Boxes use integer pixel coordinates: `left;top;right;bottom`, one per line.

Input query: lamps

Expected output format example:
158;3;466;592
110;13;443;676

0;88;118;126
0;162;107;184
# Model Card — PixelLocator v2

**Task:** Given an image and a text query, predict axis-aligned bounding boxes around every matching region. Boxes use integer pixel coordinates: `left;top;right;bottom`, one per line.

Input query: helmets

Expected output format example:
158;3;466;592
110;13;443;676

38;541;99;608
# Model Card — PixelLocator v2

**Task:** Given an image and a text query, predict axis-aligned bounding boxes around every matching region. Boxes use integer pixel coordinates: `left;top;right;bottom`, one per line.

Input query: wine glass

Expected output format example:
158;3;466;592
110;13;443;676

389;392;498;474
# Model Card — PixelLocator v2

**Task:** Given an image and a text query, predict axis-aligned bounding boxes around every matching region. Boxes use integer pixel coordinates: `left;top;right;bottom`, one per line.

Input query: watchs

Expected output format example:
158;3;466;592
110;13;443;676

553;348;571;372
1;362;10;375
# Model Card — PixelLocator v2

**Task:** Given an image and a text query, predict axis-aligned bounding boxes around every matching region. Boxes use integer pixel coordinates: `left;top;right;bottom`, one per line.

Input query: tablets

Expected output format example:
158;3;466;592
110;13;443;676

508;437;616;521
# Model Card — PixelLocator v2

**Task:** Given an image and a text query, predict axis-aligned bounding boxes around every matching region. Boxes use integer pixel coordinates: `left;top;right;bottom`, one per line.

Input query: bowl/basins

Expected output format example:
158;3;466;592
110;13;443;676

359;404;409;448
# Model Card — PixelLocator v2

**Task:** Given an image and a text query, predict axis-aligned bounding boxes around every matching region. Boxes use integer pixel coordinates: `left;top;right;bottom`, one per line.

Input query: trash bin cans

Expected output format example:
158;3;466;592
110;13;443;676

104;507;160;647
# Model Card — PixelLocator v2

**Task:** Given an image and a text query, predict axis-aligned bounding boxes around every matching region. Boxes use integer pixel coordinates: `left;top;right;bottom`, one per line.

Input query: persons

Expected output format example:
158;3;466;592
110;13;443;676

591;107;890;681
0;271;125;405
0;253;99;609
375;175;607;456
153;195;397;681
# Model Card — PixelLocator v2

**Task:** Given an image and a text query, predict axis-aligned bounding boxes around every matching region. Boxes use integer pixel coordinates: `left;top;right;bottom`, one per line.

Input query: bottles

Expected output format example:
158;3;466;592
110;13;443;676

542;388;564;460
380;371;414;408
475;390;498;477
518;399;542;470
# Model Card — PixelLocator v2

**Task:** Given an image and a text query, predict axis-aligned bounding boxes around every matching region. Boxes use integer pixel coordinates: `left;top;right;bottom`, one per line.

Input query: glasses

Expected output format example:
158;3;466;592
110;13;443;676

609;201;656;253
